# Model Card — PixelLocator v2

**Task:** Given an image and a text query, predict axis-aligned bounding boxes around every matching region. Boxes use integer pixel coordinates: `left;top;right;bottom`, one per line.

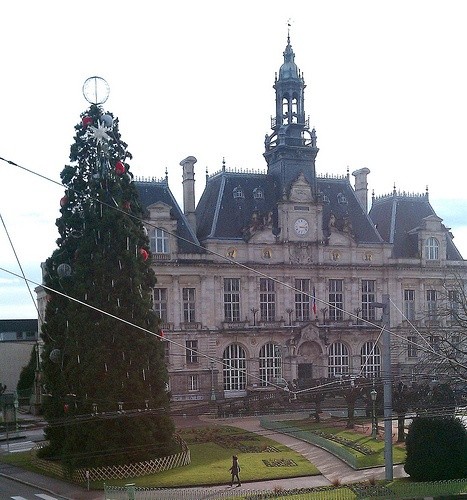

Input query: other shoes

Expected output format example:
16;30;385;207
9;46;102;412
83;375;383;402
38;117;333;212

236;484;241;487
229;483;232;487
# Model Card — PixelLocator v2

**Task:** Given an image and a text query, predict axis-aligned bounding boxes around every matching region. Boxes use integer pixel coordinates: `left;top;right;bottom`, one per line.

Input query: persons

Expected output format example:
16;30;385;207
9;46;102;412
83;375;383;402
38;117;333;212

280;377;307;405
228;455;241;489
